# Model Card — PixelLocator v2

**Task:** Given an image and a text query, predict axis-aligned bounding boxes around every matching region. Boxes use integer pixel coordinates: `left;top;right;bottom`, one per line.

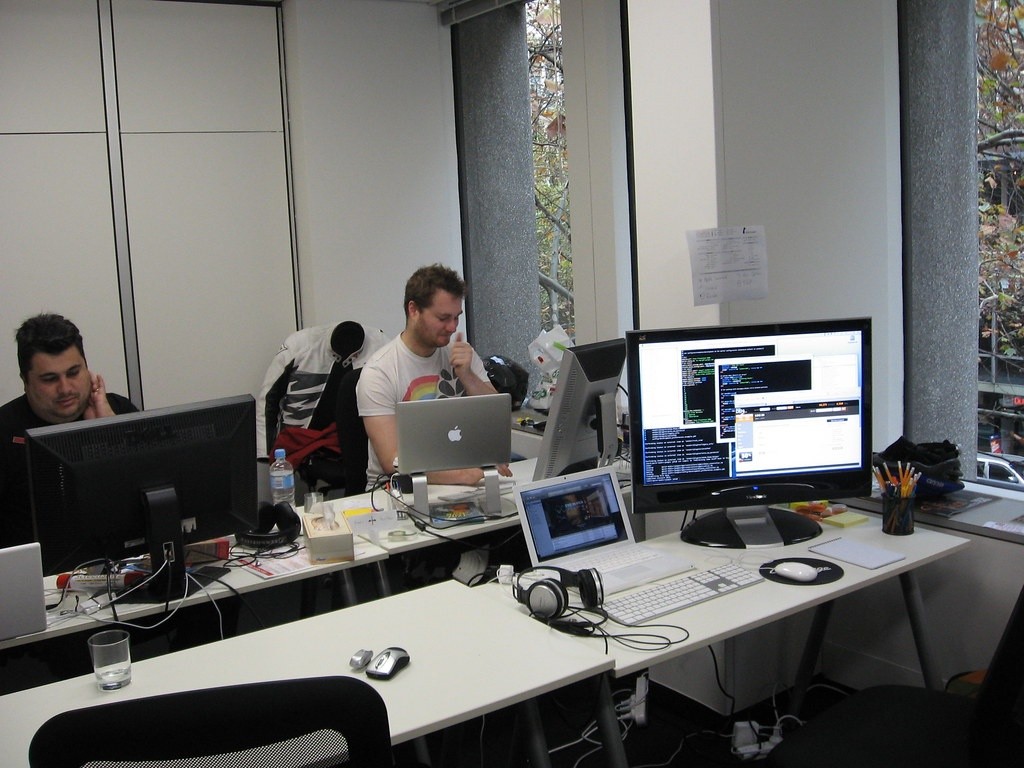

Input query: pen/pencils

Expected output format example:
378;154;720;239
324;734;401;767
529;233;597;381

871;461;922;534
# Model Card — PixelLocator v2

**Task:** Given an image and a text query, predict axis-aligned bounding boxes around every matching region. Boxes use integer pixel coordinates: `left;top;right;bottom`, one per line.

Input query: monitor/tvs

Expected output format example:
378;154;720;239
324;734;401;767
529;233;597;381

626;317;872;549
25;394;258;604
530;338;626;482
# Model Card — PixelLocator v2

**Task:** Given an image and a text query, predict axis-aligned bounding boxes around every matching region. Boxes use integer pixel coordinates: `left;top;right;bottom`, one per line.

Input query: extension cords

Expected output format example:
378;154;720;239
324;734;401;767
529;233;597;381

738;741;776;761
636;676;647;727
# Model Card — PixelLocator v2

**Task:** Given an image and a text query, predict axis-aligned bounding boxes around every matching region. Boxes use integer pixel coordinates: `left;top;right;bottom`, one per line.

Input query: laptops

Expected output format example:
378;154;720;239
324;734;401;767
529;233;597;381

0;542;47;640
512;465;696;596
393;393;512;475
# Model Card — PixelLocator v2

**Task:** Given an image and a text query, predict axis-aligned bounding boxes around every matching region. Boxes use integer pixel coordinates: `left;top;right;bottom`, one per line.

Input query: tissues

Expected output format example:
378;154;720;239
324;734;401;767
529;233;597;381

301;503;355;564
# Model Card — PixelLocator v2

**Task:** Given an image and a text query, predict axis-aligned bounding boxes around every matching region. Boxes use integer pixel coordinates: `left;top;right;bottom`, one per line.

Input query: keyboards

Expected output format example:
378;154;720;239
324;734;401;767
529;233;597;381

599;563;764;627
437;481;518;503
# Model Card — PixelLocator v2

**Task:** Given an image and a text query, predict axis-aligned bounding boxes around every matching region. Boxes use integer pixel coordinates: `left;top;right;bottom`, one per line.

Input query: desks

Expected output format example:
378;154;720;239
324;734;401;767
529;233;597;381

0;508;388;651
471;500;970;768
298;453;713;598
0;579;633;768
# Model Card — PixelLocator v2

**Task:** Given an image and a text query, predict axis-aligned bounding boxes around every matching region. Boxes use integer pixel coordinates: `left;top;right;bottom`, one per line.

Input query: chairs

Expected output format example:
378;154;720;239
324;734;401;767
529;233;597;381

336;366;368;500
29;676;393;768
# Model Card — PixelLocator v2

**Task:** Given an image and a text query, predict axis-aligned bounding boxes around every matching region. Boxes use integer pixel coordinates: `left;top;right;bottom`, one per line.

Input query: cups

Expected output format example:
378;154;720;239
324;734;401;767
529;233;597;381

87;630;131;691
882;493;916;535
303;492;324;515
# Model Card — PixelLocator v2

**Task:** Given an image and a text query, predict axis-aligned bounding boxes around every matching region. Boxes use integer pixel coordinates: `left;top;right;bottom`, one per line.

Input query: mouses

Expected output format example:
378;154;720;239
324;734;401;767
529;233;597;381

366;647;409;680
775;562;818;582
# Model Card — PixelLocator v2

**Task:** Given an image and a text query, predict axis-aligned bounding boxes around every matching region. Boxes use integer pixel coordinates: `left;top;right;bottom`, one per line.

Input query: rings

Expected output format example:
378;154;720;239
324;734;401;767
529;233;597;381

94;388;100;392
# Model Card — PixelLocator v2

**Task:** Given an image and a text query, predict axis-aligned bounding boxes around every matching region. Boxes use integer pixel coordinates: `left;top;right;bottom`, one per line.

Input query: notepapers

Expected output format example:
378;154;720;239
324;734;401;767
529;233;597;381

821;511;869;528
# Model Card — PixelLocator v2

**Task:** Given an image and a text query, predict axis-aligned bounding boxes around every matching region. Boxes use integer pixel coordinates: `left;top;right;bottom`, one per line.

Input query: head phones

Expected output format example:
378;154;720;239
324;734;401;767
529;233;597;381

513;565;603;619
236;501;301;548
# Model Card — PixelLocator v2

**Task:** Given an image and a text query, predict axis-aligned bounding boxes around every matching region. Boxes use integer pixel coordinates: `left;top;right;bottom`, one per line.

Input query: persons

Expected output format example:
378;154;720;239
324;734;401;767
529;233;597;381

0;314;239;685
356;265;514;601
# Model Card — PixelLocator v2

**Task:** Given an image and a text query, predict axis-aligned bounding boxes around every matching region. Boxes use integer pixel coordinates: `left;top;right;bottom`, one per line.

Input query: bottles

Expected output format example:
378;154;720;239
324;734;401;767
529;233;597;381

269;449;296;512
